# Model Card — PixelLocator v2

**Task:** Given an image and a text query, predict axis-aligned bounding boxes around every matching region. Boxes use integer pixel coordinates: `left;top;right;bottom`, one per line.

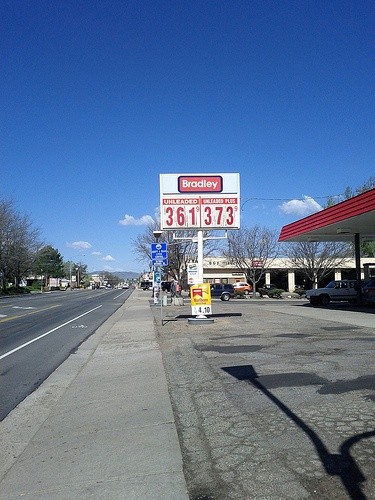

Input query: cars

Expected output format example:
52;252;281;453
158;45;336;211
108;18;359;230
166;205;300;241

305;279;366;306
232;282;253;295
355;277;375;308
122;283;129;289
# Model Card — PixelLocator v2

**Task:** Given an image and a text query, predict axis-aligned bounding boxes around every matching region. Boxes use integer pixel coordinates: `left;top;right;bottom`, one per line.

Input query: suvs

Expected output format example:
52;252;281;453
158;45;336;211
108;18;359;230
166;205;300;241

210;283;236;301
